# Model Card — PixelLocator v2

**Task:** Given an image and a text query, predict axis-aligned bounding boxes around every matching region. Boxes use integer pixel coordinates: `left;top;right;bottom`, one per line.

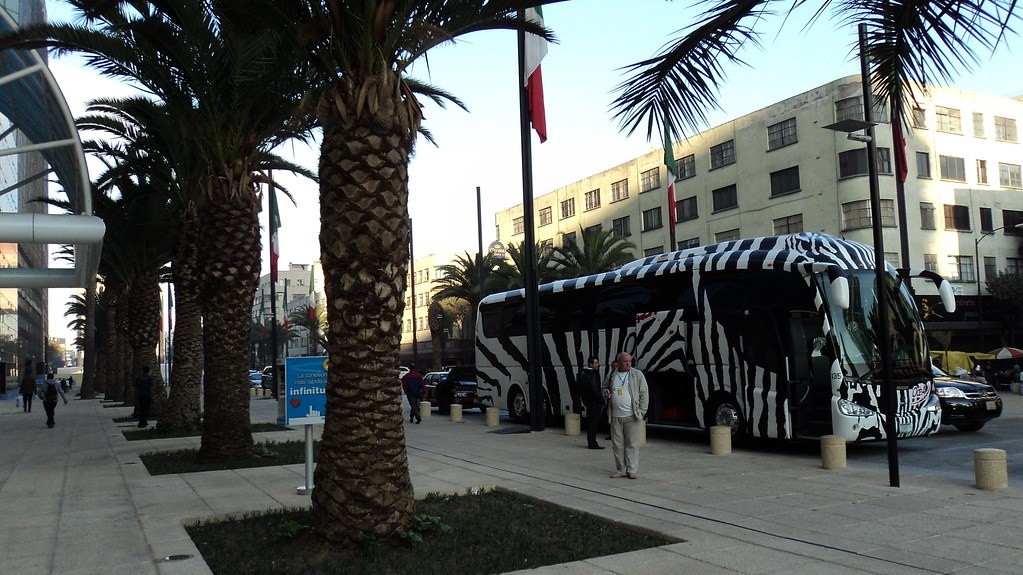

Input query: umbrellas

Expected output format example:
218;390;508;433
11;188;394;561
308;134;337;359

988;347;1023;359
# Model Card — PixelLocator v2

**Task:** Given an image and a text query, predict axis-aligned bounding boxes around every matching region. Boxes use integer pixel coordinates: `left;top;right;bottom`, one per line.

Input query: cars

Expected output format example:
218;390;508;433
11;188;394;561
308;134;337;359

930;356;1004;431
422;364;477;411
249;365;273;390
398;366;411;380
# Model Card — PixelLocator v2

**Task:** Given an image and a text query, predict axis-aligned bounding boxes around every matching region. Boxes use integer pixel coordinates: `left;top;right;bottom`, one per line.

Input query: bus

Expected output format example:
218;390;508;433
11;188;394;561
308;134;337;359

474;231;958;449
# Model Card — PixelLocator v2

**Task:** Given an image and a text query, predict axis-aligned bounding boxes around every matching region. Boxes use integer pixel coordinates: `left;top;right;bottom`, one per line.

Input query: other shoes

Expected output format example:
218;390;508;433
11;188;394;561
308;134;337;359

588;441;605;449
604;435;611;440
409;418;413;423
627;472;637;478
610;471;626;478
415;419;422;425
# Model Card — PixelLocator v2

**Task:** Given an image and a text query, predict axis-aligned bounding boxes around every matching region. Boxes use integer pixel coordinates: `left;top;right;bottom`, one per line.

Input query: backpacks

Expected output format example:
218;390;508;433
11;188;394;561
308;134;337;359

44;380;59;404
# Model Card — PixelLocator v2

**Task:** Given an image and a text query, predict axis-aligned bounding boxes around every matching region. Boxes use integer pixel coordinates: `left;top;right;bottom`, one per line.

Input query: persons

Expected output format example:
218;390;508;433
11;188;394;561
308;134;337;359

60;378;67;393
18;368;38;412
401;363;426;424
601;352;649;480
66;376;75;390
1011;361;1020;383
580;357;606;449
603;361;617;440
847;313;860;332
38;373;68;428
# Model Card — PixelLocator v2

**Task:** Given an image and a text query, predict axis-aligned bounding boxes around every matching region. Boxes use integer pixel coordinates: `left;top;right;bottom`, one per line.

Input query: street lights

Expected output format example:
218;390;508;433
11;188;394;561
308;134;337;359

975;222;1023;351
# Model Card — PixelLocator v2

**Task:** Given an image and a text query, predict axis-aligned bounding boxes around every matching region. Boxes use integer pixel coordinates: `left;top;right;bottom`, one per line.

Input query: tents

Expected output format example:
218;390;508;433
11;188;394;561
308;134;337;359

930;351;995;376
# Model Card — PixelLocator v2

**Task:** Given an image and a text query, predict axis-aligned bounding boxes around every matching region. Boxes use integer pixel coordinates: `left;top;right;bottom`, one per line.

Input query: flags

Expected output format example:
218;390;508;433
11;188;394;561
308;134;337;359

270;182;281;284
663;114;680;222
258;293;266;339
309;267;316;321
169;287;174;330
526;4;548;144
159;298;163;332
282;283;288;333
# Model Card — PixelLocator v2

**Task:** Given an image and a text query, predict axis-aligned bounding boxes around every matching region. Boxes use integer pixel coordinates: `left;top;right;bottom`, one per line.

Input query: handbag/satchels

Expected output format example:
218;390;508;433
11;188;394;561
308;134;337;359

16;394;23;408
